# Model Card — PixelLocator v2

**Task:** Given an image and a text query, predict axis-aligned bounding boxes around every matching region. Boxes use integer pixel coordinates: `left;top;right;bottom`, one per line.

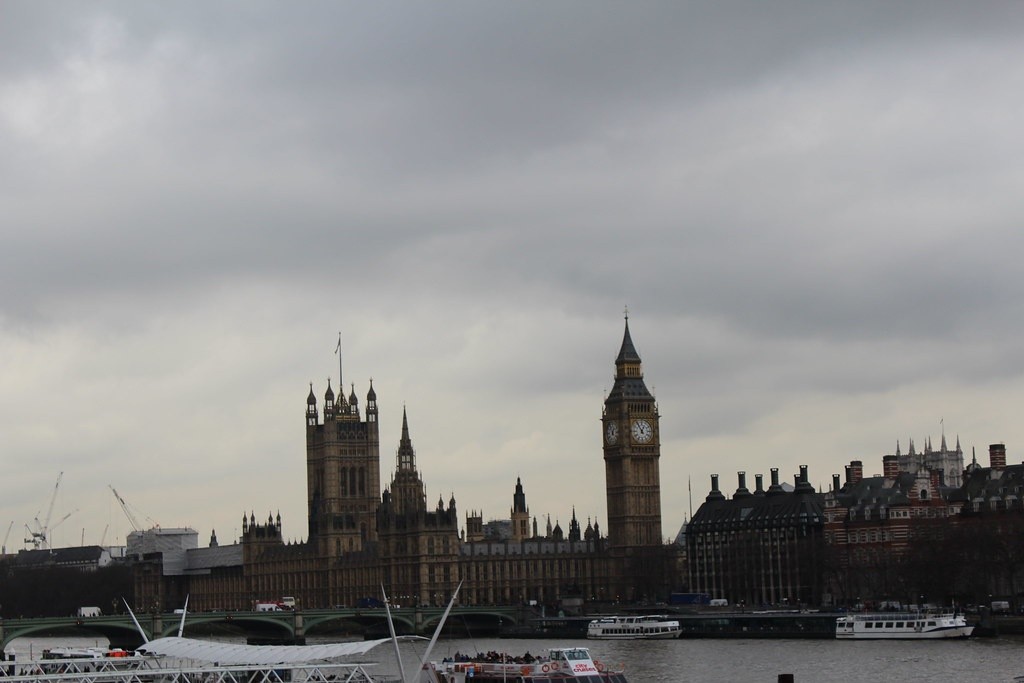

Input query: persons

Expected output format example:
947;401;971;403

441;650;550;664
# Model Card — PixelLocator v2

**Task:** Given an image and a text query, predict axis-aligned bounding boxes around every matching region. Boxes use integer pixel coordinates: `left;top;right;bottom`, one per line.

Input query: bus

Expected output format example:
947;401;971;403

252;596;295;609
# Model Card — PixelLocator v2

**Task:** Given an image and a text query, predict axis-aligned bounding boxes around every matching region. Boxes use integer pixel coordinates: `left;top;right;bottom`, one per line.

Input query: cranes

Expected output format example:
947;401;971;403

39;469;64;549
105;482;161;558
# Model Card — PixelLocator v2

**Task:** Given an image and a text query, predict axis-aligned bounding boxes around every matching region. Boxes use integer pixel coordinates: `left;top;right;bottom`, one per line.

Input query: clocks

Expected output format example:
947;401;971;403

632;420;652;443
607;423;618;443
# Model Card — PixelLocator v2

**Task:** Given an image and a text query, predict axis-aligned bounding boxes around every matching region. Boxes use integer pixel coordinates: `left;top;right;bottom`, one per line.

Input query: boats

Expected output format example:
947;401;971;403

37;649;165;683
586;615;682;639
835;609;975;639
424;647;627;683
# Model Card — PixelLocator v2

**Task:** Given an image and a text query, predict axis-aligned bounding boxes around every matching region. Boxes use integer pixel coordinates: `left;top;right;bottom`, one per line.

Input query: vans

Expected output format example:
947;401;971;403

175;609;189;613
80;607;101;616
709;600;728;607
256;603;282;611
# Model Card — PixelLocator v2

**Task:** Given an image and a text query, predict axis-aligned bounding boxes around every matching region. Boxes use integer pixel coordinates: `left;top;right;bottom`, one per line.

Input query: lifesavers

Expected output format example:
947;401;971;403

542;664;549;672
593;659;598;664
551;661;559;670
596;663;604;671
